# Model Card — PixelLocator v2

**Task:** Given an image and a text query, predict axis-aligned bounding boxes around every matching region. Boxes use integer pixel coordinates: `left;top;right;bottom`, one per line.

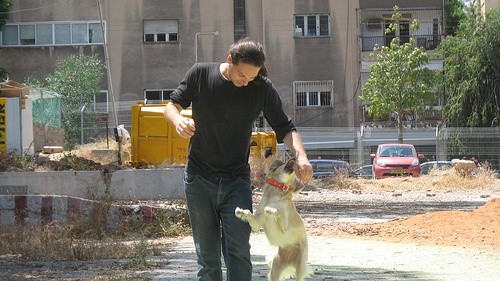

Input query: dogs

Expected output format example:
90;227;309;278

234;157;317;281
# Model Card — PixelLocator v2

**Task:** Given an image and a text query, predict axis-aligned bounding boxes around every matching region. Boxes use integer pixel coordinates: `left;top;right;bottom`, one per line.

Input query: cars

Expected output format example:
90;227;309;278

421;161;453;174
351;165;374;178
370;143;424;179
309;158;349;179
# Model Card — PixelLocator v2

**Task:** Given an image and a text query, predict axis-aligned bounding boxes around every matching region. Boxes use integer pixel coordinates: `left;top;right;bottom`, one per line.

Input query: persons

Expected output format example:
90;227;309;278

163;40;313;281
